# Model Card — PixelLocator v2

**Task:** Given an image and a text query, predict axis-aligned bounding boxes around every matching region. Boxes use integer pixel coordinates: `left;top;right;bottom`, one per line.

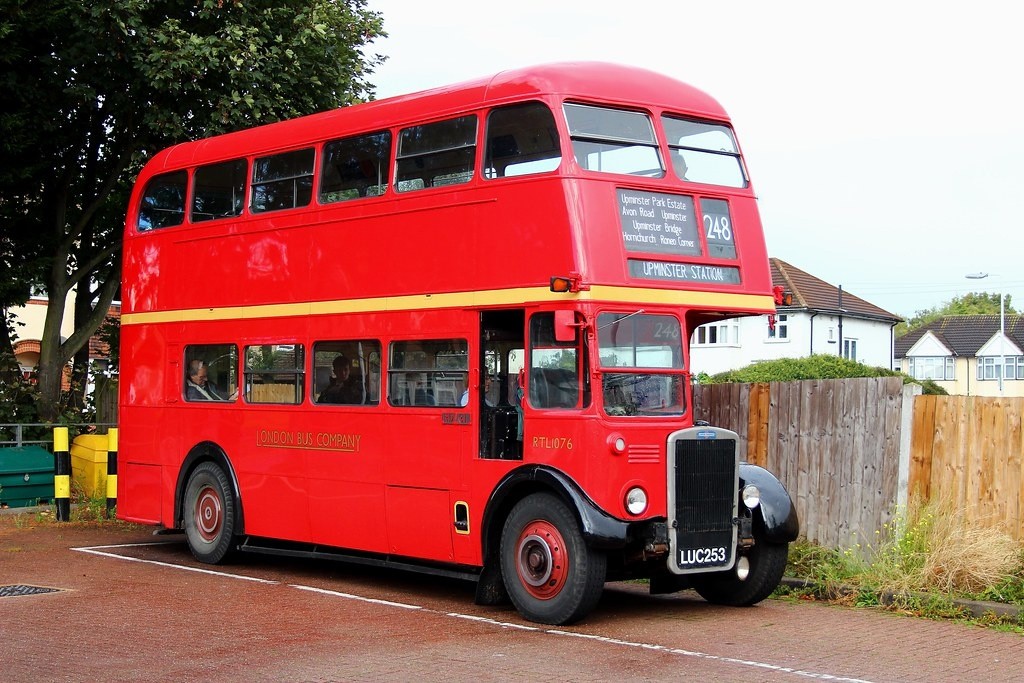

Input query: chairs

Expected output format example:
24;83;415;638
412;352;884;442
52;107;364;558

535;368;577;409
263;375;275;384
315;366;332;393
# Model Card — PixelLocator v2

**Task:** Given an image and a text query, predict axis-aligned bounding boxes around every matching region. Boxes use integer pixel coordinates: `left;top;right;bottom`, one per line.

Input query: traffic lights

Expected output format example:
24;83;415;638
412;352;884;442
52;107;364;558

782;292;794;307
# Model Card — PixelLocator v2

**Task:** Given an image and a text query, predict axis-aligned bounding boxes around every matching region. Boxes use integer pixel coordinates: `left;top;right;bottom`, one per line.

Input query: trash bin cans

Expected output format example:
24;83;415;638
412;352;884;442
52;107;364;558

70;434;109;499
0;445;55;508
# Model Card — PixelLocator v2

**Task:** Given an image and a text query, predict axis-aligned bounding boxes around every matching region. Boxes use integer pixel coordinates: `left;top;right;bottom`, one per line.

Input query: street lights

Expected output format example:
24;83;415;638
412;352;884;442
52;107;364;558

965;271;1006;398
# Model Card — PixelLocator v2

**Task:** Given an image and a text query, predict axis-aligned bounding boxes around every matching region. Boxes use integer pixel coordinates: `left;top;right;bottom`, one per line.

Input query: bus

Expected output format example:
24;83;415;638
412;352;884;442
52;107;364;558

116;61;801;627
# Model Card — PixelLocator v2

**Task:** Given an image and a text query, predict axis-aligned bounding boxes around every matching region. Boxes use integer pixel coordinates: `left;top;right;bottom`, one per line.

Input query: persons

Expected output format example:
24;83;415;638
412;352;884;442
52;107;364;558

401;373;437;406
460;366;497;407
186;360;239;401
318;355;368;405
